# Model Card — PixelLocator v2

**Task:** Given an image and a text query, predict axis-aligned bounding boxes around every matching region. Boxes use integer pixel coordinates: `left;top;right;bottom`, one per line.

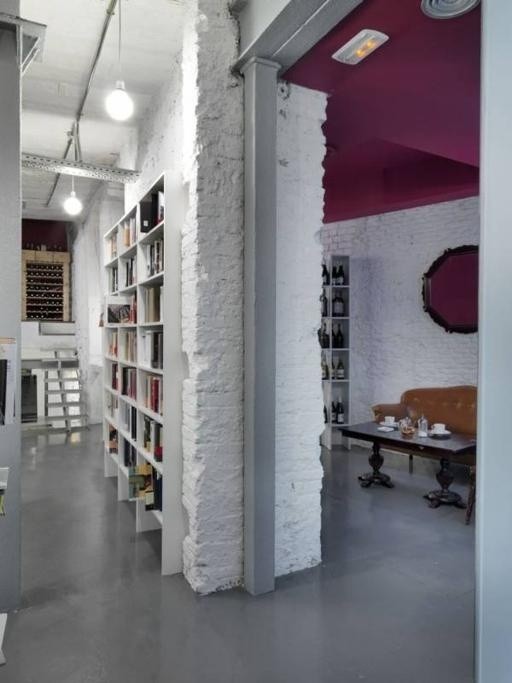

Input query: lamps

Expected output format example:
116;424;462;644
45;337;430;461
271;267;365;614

100;1;137;122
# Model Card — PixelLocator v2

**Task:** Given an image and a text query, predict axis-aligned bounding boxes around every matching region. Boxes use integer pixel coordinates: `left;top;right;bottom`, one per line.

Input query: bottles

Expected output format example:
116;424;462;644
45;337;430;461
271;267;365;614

332;398;344;423
320;288;327;316
417;417;428;438
324;323;344;348
332;356;345;380
333;290;344;316
323;264;345;285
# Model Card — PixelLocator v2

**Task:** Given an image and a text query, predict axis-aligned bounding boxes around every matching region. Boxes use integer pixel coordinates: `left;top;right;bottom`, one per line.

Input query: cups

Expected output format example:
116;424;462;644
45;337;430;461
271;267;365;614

385;415;395;423
431;423;446;431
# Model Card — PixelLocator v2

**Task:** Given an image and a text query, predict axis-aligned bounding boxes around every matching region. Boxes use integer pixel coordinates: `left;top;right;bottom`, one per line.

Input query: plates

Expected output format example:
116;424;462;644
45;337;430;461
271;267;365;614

428;430;452;438
380;421;400;428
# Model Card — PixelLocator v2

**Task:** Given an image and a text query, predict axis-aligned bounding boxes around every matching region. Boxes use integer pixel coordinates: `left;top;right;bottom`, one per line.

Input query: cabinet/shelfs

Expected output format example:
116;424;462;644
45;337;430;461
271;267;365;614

320;252;352;452
26;258;65;322
101;171;185;577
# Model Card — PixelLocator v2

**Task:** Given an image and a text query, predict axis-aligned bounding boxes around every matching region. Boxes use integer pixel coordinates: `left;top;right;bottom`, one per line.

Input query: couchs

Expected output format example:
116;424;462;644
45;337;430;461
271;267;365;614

369;385;476;482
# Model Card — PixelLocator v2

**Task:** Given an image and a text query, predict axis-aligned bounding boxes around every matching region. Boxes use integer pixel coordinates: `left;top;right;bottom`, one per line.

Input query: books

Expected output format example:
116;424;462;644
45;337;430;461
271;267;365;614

102;189;162;514
